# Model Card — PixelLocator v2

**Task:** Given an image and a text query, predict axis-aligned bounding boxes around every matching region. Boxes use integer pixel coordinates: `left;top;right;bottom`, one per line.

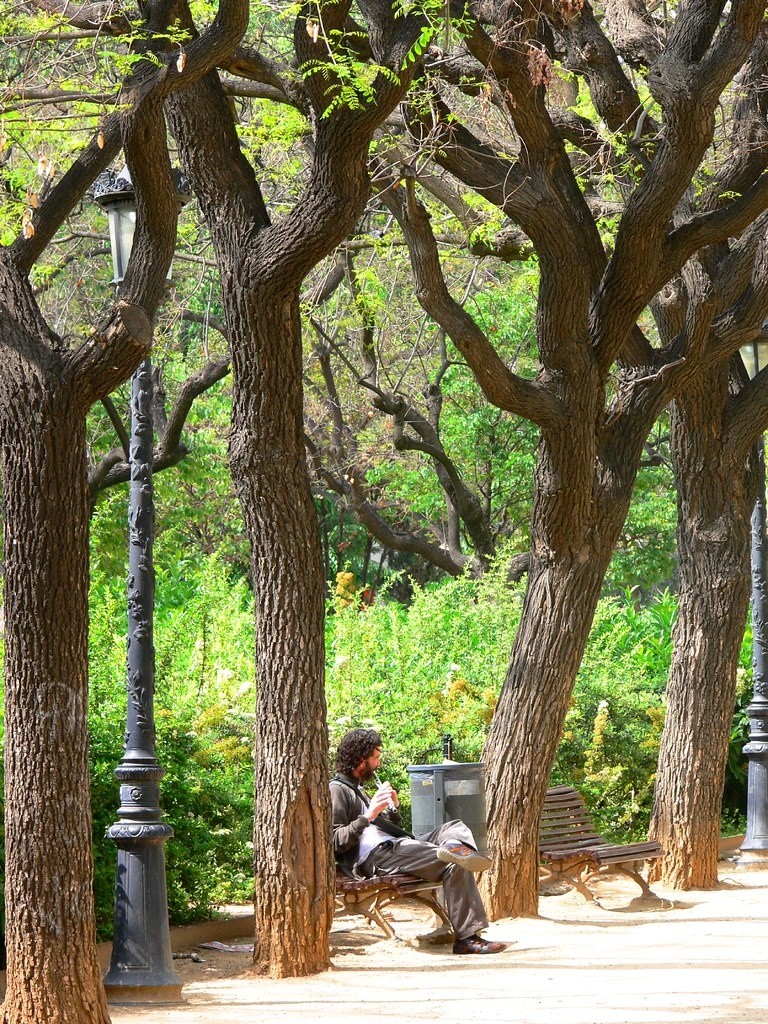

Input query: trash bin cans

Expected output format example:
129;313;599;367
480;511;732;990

406;762;490;856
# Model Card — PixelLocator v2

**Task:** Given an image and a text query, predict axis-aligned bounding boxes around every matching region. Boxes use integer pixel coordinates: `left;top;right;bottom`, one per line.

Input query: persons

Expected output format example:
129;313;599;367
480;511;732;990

329;729;508;955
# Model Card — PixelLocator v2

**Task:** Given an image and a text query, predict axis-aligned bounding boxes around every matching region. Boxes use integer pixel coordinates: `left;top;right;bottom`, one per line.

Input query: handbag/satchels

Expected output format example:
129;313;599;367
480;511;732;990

374;815;414;840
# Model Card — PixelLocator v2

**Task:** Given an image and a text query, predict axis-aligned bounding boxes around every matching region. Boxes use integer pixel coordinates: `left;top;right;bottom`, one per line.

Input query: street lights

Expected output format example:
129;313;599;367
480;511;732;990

92;160;186;1009
737;318;768;868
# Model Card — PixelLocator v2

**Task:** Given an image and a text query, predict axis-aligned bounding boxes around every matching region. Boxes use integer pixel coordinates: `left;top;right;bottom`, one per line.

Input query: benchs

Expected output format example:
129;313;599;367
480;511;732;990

537;783;666;907
333;864;450;942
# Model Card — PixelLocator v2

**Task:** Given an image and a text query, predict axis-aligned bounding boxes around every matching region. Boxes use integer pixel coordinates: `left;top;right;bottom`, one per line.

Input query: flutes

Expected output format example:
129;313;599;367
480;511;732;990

374;772;398;813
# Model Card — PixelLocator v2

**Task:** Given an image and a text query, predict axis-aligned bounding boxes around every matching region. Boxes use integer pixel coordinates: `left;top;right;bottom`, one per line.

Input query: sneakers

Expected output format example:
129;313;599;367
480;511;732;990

436;844;490;871
454;934;506;954
